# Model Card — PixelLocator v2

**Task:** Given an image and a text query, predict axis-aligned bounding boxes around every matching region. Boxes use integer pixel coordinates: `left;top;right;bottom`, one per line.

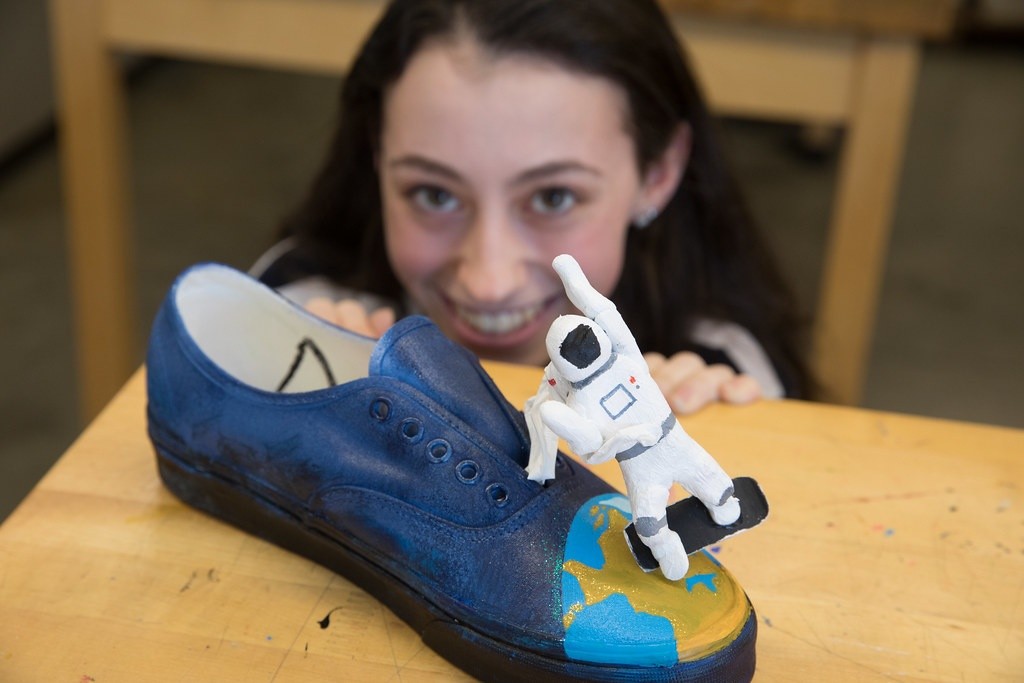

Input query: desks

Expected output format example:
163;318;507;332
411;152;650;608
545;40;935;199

0;355;1024;683
53;0;965;427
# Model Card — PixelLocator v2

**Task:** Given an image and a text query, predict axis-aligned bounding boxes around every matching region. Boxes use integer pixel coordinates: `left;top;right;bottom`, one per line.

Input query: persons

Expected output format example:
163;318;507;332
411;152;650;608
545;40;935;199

265;0;814;418
541;254;742;582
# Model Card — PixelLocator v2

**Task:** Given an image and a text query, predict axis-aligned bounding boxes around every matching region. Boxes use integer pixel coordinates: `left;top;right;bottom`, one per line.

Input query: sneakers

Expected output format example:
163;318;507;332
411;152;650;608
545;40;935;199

147;264;757;683
